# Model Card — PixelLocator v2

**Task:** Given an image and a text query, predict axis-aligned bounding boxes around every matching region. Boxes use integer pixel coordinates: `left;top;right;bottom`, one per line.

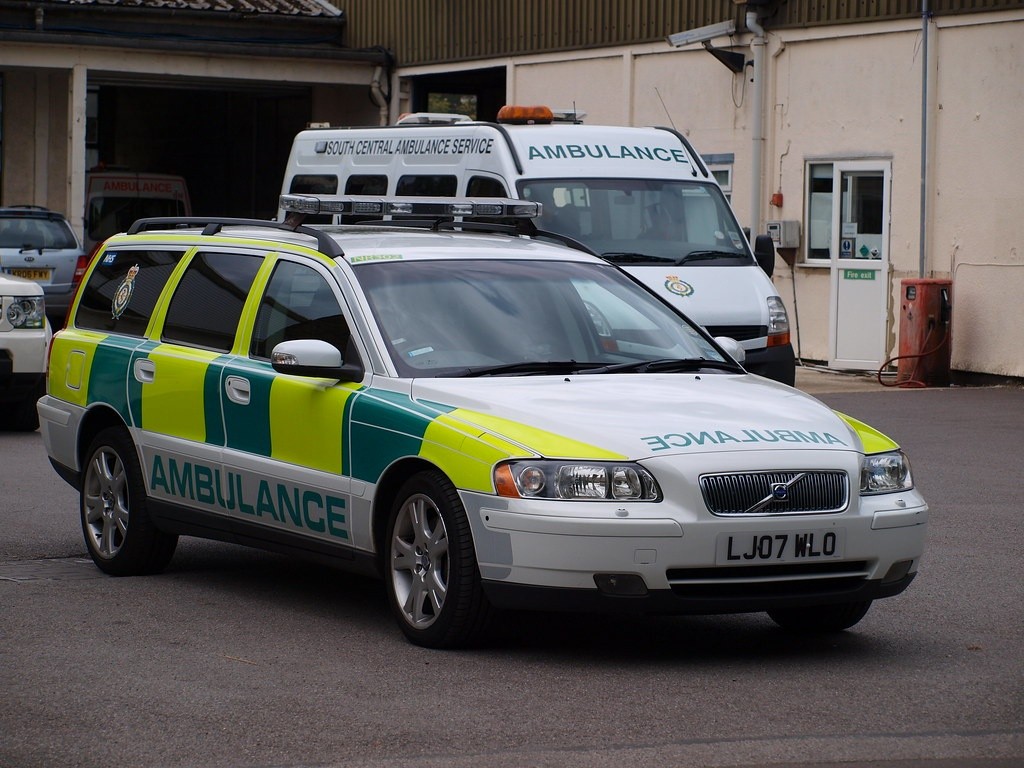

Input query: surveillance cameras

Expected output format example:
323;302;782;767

662;18;736;47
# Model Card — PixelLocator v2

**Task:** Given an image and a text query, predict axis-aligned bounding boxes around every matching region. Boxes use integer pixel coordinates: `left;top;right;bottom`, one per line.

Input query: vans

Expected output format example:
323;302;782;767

86;169;193;274
269;99;799;385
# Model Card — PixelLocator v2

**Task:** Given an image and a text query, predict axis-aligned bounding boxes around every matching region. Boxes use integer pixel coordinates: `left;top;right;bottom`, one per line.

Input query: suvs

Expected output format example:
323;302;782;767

34;215;926;648
1;202;86;320
1;270;58;434
